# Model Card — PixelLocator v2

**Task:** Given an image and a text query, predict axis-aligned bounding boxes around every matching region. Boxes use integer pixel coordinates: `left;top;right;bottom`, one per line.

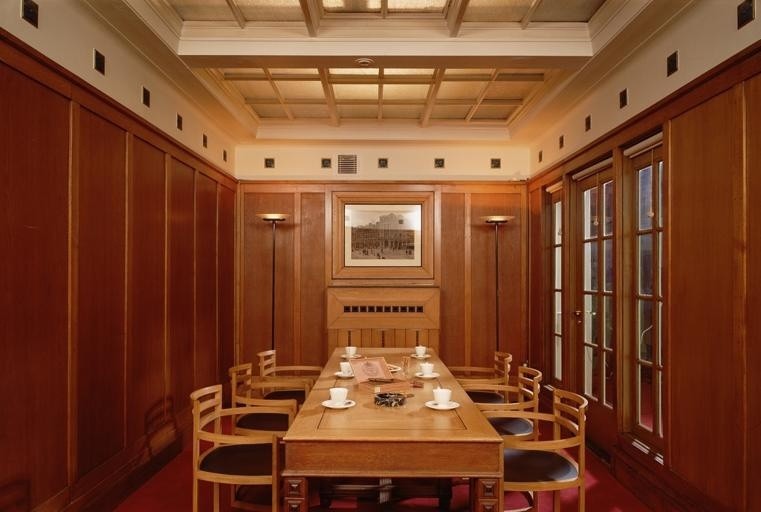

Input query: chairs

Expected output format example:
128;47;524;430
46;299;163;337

190;385;292;512
460;366;542;511
256;351;322;409
480;389;587;512
445;352;512;394
228;363;310;511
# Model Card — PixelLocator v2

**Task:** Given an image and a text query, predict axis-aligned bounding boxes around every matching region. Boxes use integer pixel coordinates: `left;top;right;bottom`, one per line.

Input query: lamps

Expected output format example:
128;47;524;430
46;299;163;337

481;215;517;353
255;212;291;351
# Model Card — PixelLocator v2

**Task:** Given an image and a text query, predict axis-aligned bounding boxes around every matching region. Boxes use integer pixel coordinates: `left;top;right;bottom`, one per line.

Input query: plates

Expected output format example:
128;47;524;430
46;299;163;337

410;353;433;360
420;363;434;375
424;401;460;411
341;354;362;359
386;363;402;372
339;362;349;373
415;346;427;355
336;371;354;378
415;373;440;379
322;400;356;410
345;347;357;354
329;388;349;404
432;388;451;403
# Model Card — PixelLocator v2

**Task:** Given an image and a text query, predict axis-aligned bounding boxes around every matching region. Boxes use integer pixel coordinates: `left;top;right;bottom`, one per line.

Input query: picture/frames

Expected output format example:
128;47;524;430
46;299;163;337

344;202;423;268
